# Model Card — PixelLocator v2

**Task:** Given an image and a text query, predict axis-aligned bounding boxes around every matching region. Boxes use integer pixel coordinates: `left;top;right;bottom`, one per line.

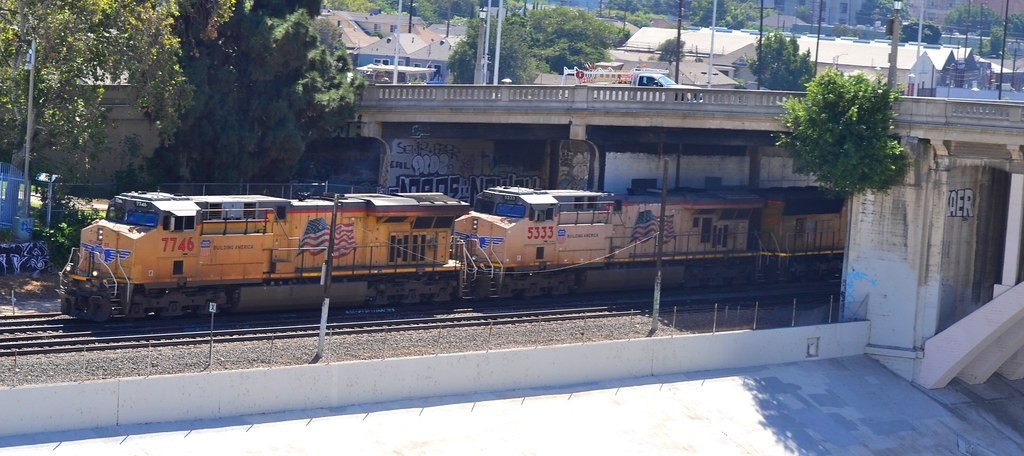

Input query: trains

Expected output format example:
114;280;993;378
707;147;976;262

54;185;848;322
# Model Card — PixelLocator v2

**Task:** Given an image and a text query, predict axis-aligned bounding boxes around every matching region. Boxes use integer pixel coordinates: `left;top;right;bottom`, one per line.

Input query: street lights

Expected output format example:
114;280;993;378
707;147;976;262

887;0;904;95
17;37;40;244
472;8;488;84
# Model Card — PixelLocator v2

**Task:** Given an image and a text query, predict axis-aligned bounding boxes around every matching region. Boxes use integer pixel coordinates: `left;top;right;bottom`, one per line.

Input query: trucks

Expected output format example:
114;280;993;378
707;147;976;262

559;65;684;101
